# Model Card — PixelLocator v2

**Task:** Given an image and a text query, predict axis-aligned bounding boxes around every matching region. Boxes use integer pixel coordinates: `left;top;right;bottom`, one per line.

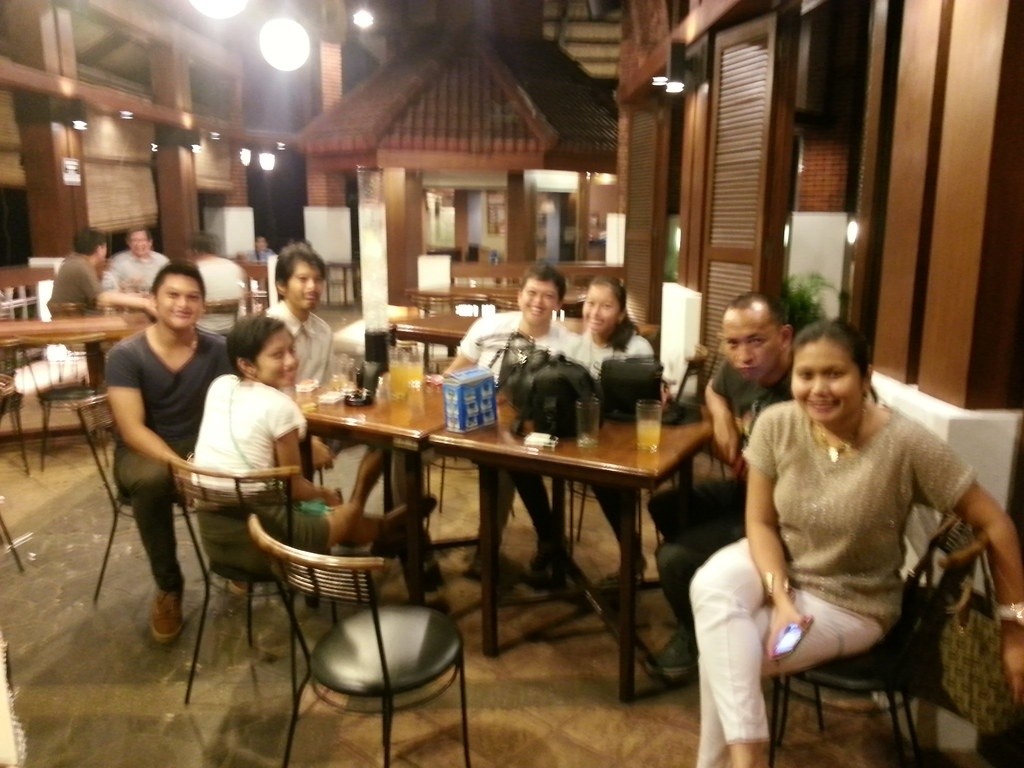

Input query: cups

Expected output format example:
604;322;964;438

405;342;425;393
389;349;409;402
634;398;663;454
577;395;600;450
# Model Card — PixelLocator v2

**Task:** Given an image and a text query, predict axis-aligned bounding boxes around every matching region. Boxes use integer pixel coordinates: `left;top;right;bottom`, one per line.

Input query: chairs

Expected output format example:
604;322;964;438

1;303;987;767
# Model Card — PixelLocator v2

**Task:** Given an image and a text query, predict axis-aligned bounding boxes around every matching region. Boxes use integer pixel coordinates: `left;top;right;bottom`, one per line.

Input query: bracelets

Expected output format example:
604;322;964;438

996;601;1024;626
763;573;791;594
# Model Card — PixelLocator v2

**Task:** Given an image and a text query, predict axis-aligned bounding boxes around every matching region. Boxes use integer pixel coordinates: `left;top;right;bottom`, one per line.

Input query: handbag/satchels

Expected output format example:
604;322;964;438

597;357;687;425
648;477;746;541
873;513;1022;736
489;333;549;419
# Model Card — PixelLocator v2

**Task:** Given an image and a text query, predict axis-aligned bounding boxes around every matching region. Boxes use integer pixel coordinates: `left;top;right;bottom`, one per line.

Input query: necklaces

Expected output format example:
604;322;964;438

815;409;864;462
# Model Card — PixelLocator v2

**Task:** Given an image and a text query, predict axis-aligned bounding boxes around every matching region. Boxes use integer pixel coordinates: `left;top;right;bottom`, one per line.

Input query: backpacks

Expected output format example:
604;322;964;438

511;355;603;438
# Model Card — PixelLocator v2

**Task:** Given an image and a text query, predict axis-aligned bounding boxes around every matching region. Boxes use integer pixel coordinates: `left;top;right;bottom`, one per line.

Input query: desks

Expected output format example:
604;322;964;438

5;316;127;391
284;361;449;609
387;317;480;362
428;400;715;705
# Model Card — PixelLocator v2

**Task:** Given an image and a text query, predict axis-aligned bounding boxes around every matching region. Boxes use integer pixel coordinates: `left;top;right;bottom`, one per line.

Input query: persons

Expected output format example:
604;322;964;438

180;232;248;299
106;223;171;293
262;240;387;509
105;261;240;643
441;262;654;592
687;319;1024;768
251;236;275;260
189;316;436;577
48;226;157;321
640;291;795;678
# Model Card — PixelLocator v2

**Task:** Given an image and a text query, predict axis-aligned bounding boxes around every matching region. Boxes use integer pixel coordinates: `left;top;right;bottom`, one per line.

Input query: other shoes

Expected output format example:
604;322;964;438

465;544;482;581
644;621;698;683
535;538;565;586
600;566;644;590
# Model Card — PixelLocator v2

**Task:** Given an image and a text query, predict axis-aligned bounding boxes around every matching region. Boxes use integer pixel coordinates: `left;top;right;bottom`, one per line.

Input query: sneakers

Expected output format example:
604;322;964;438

151;584;184;643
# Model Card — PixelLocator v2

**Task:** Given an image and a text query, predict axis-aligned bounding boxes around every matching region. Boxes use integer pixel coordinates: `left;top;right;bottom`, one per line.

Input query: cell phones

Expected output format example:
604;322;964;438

770;616;815;661
